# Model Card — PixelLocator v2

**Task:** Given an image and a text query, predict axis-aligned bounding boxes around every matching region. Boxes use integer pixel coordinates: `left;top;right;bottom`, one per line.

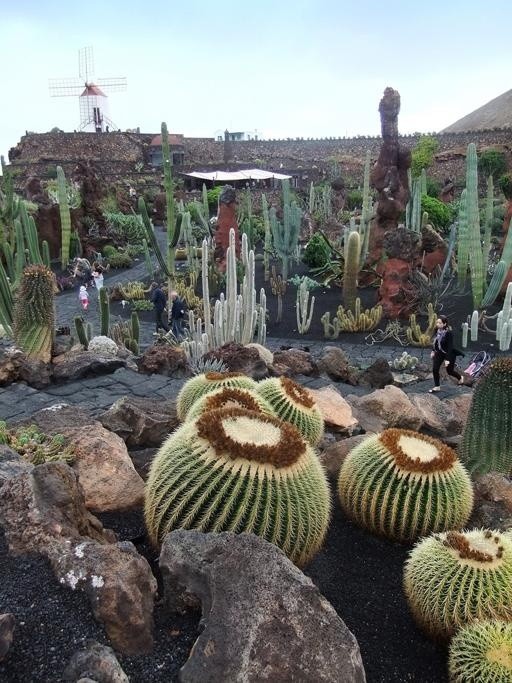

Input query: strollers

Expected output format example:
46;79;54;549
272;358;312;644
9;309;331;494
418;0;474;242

463;350;497;383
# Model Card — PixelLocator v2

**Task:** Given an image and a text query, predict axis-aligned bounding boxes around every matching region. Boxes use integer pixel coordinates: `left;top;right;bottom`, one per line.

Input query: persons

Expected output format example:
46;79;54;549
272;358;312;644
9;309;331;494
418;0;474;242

91;268;104;300
429;316;464;393
148;282;170;336
169;291;187;340
79;284;90;311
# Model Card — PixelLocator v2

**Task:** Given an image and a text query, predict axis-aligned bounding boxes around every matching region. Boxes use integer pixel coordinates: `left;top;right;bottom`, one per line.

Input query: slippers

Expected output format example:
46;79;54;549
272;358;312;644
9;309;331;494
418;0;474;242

428;388;441;393
457;381;464;388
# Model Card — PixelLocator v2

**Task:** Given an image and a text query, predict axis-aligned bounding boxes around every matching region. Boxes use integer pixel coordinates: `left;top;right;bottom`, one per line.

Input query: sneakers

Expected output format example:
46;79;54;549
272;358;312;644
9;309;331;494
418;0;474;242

153;332;160;336
